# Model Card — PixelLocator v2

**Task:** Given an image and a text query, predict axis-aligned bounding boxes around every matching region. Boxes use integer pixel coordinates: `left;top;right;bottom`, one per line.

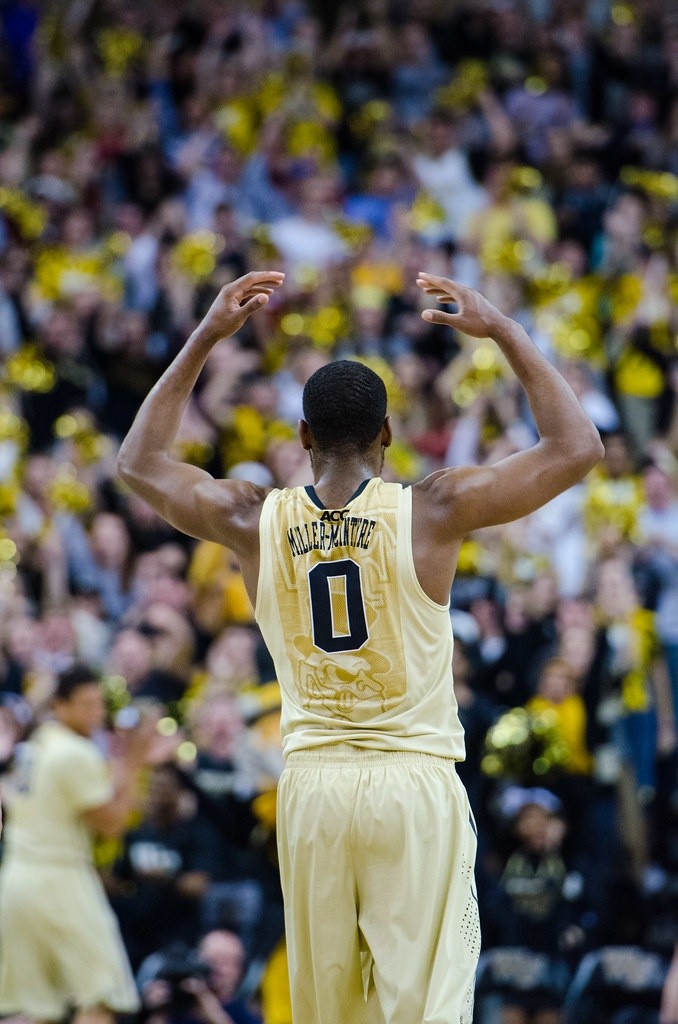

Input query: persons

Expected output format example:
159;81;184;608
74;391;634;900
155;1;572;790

115;270;606;1024
0;0;675;1023
0;663;141;1023
